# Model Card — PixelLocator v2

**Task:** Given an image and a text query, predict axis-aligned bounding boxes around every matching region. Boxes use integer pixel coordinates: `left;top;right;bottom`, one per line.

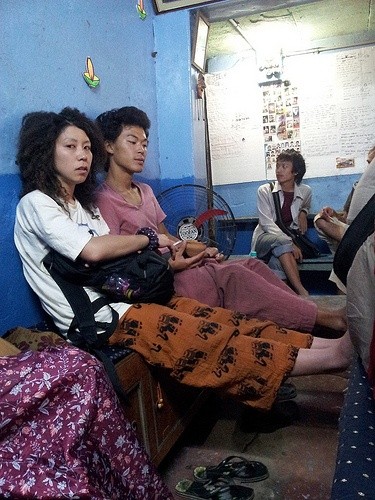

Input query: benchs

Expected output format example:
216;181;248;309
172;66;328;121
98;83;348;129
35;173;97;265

226;253;334;271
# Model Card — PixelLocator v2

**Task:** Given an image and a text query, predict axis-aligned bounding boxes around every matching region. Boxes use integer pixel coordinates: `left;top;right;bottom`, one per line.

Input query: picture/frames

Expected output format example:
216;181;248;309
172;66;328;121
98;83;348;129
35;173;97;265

192;10;211;73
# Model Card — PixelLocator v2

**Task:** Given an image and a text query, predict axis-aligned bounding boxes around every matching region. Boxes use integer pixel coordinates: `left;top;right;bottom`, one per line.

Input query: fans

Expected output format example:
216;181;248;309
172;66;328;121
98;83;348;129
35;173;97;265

157;184;236;259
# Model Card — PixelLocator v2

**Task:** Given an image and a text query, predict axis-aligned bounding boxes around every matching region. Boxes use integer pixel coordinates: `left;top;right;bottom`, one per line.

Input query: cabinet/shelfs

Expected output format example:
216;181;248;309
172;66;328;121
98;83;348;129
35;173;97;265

113;351;207;470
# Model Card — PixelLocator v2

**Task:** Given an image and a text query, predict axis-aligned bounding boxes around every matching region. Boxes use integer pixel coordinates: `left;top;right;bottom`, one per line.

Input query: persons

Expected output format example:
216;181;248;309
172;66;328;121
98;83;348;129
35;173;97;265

93;107;348;339
249;148;313;297
12;107;355;411
314;144;375;500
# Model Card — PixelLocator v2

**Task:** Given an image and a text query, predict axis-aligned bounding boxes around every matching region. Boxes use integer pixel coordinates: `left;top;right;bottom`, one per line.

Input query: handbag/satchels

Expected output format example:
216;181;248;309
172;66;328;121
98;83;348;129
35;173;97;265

44;244;175;305
291;229;320;259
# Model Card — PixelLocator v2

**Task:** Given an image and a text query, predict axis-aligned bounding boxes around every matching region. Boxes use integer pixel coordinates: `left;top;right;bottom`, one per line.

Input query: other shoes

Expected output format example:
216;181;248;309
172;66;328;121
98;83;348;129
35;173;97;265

278;383;297;400
236;401;297;432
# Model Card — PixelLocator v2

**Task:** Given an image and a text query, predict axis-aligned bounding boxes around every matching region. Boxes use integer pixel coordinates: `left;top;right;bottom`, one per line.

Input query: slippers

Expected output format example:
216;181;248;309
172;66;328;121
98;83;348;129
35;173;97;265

193;455;269;483
174;480;255;500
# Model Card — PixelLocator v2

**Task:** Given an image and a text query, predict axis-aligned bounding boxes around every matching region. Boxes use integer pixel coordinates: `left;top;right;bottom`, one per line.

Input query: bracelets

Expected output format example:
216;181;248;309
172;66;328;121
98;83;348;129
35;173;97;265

136;227;160;252
